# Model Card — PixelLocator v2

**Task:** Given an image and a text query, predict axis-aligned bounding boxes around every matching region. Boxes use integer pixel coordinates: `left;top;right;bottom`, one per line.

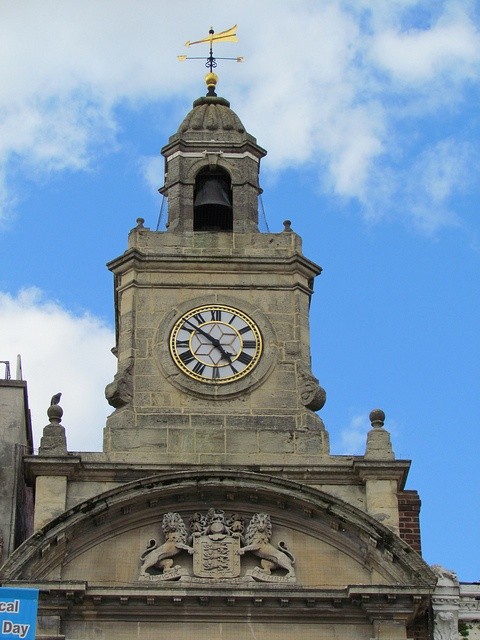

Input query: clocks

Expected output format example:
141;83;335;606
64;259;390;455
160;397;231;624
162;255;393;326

152;293;280;402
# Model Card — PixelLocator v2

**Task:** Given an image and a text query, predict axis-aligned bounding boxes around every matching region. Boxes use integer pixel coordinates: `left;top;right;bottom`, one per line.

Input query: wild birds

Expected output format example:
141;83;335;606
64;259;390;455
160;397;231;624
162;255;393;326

50;393;62;405
